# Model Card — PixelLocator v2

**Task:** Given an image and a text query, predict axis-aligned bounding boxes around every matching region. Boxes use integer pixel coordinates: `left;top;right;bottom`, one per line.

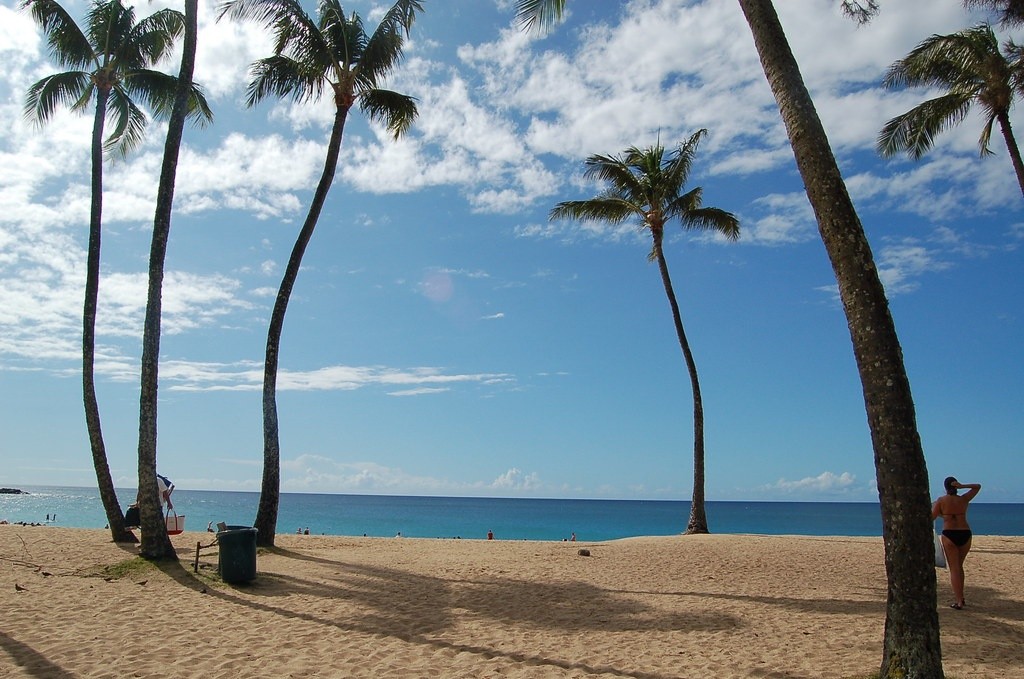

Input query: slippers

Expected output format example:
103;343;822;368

950;597;966;609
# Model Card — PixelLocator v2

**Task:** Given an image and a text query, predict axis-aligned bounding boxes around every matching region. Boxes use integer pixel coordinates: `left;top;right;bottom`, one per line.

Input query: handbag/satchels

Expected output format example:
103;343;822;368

933;529;947;569
164;508;186;535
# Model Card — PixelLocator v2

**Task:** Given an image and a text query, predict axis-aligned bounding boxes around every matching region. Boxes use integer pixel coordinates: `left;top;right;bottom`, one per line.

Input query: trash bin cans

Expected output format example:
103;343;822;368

216;525;258;587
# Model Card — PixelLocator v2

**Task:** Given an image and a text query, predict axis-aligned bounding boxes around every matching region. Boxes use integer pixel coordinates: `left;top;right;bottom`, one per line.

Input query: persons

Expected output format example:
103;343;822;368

46;512;56;523
571;533;575;541
933;477;981;609
128;477;172;548
488;530;493;540
297;527;309;535
396;531;401;538
